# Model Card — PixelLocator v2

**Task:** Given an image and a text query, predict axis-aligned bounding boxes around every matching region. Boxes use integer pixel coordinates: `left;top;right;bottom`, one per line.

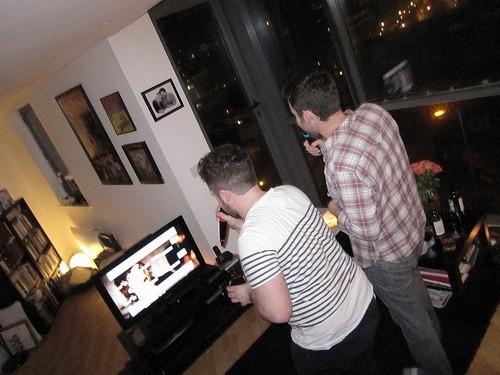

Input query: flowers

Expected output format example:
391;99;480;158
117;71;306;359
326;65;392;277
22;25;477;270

410;160;443;187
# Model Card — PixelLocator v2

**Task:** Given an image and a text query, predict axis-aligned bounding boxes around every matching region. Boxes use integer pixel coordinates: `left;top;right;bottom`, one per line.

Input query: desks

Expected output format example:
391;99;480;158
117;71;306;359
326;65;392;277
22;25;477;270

419;213;492;310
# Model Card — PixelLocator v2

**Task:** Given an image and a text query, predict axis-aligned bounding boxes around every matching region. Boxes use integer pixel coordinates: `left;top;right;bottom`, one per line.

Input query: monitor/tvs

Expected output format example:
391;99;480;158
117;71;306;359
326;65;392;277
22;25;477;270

90;215;209;332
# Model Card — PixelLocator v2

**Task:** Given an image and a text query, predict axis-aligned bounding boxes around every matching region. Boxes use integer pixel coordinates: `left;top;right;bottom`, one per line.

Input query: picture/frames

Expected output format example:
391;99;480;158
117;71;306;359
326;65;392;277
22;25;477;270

54;84;133;185
140;78;185;123
121;140;165;184
100;90;138;136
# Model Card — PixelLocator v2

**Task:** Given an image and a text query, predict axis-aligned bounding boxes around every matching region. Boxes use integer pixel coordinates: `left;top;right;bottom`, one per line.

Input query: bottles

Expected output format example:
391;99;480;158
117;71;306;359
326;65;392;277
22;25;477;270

427;198;445;237
447;181;465;218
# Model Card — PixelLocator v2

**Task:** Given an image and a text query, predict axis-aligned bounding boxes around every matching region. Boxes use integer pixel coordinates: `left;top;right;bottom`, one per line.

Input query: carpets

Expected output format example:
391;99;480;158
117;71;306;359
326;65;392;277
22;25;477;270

224;237;500;375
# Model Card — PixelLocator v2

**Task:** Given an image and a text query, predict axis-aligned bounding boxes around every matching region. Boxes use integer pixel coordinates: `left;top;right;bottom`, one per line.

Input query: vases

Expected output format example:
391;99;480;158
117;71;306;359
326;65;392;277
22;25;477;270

419;184;441;211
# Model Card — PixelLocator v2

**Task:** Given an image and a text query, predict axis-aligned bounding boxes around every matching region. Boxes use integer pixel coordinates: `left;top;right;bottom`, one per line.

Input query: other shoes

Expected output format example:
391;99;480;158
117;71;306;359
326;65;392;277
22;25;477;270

402;367;418;375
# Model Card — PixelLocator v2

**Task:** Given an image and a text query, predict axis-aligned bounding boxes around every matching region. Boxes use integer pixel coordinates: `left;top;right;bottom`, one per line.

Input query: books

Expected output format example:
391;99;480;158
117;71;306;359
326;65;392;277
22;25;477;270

0;215;67;319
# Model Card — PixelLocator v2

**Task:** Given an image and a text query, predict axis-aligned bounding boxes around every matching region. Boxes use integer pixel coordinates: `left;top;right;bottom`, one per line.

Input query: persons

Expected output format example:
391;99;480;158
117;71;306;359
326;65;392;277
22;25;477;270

196;144;381;375
152;88;177;113
281;68;454;375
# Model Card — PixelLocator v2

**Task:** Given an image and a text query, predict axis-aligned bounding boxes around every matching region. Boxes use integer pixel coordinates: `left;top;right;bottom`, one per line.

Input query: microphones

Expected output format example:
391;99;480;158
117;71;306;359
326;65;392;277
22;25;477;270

219;208;228;246
302;131;324;160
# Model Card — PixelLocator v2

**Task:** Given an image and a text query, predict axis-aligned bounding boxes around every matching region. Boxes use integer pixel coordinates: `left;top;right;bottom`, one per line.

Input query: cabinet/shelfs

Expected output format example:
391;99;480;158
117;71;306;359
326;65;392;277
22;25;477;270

0;197;67;336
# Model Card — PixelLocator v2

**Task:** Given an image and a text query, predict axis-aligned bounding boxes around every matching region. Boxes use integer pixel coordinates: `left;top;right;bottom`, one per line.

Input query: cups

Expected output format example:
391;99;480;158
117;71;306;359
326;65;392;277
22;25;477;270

441;235;456;252
229;275;251;306
